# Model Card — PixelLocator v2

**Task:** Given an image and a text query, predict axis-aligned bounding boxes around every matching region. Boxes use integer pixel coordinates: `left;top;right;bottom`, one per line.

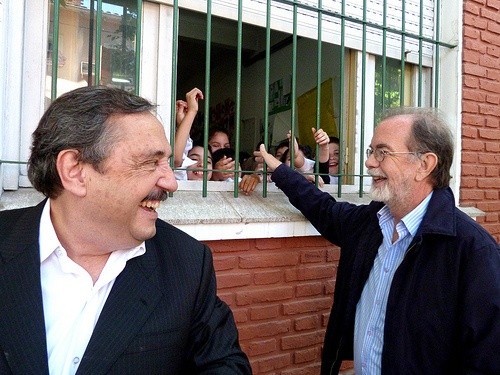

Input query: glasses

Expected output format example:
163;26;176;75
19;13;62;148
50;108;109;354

366;148;429;161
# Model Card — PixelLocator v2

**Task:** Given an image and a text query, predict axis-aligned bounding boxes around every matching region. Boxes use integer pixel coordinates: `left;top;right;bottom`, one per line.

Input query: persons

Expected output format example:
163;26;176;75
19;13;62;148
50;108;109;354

173;76;340;196
254;106;500;375
0;81;254;375
